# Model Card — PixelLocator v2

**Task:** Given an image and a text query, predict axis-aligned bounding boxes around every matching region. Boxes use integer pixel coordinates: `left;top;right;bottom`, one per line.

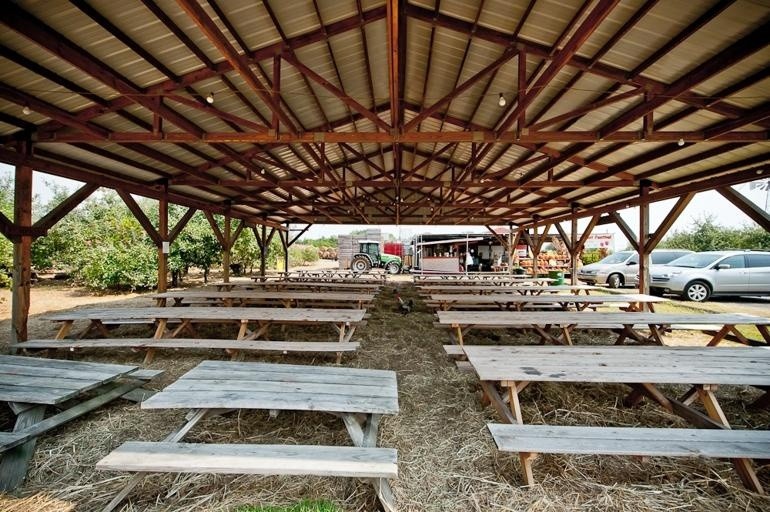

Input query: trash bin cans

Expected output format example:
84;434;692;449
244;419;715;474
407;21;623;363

548;270;564;293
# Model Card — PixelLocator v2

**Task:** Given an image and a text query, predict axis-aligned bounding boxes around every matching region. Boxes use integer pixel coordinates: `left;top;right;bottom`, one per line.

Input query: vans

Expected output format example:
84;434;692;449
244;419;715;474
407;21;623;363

576;248;692;289
632;249;770;303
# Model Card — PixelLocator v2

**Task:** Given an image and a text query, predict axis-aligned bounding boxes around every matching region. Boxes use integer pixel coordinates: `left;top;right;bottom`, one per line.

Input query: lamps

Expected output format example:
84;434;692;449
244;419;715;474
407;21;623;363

678;135;685;146
22;100;31;115
498;92;506;106
206;91;214;104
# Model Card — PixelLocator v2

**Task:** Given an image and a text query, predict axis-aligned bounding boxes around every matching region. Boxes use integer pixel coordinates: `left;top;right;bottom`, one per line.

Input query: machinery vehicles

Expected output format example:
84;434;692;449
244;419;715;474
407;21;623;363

350;240;402;275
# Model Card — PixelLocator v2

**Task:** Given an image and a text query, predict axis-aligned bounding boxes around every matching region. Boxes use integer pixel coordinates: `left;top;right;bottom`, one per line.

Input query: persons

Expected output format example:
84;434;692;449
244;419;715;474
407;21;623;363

460;248;493;272
